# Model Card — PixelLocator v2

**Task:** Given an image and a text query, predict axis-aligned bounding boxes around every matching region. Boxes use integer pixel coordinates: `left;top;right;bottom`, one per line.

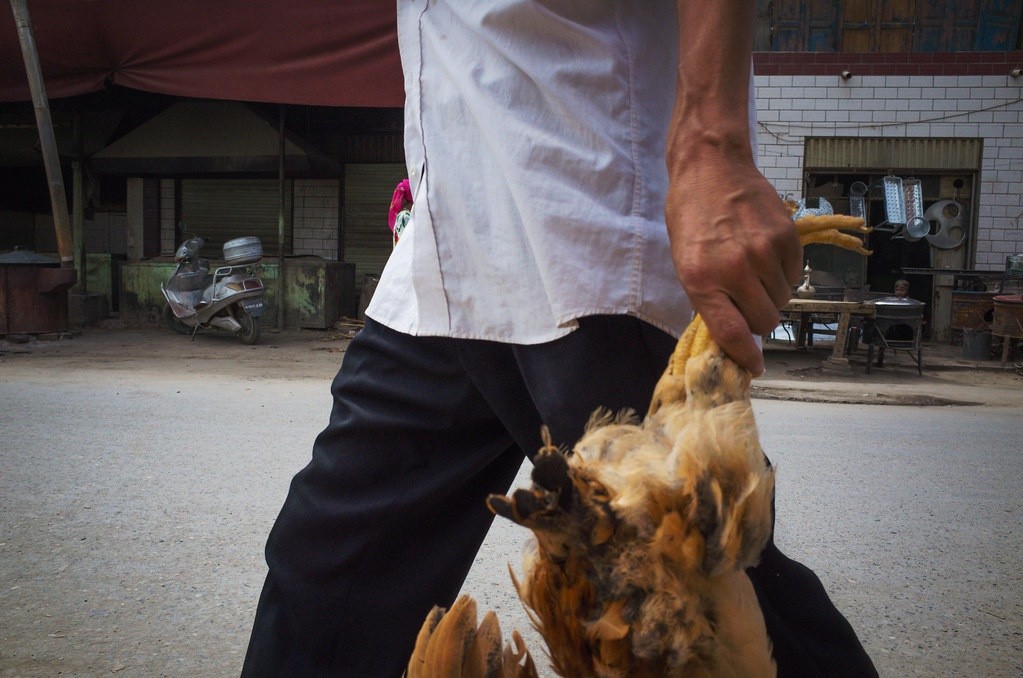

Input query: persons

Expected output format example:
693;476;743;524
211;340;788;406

241;0;878;678
895;279;910;297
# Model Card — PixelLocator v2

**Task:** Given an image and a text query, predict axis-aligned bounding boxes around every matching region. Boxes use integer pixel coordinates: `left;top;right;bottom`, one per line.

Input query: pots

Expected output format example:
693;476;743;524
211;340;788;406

866;296;926;307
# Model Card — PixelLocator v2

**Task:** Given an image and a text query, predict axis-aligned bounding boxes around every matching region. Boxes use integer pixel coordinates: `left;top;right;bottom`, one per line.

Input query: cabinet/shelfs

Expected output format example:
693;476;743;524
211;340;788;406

119;258;357;328
48;254;131;311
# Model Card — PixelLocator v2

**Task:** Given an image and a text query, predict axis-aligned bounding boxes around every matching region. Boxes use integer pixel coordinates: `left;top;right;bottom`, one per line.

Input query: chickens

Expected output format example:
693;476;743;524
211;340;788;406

400;200;874;678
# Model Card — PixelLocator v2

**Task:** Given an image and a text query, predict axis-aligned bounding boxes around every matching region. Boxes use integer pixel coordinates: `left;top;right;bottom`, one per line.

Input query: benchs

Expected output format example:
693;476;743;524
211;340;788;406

773;284;870;355
782;299;874;357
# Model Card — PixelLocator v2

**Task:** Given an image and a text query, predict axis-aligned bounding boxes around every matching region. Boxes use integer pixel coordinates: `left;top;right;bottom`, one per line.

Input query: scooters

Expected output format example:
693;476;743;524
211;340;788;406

161;221;264;345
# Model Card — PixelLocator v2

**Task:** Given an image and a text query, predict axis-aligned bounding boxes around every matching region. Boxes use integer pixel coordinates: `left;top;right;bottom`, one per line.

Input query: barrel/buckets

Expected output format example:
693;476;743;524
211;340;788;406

960;331;992;361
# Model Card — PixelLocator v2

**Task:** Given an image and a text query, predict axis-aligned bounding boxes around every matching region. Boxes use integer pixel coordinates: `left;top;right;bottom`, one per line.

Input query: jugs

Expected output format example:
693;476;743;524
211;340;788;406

796;260;815;299
